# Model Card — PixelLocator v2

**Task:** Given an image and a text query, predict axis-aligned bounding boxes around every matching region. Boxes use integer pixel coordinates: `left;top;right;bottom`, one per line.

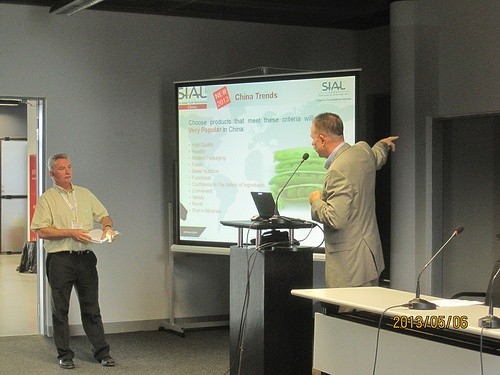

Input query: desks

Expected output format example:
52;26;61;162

291;286;500;375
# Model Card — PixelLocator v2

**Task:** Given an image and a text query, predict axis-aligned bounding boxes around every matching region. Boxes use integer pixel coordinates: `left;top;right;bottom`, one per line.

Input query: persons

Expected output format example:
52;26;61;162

309;112;399;288
30;154;116;369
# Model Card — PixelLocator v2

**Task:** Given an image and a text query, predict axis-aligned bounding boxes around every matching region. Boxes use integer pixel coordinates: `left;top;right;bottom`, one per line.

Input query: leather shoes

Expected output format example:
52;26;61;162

100;356;114;365
59;359;74;368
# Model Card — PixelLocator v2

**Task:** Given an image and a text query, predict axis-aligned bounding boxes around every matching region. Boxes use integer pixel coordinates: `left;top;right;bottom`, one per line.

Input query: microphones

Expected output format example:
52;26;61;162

408;225;465;310
271;153;309;224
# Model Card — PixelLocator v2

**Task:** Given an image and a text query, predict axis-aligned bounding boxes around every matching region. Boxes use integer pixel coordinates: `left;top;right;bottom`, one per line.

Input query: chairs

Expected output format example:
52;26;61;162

450;264;500;308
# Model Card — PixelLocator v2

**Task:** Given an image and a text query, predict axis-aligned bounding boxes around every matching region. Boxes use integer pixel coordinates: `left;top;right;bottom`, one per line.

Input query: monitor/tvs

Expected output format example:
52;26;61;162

251;191;280;219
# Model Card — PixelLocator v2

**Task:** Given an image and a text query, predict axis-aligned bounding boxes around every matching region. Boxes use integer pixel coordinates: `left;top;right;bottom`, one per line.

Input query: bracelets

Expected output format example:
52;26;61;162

104;225;111;228
